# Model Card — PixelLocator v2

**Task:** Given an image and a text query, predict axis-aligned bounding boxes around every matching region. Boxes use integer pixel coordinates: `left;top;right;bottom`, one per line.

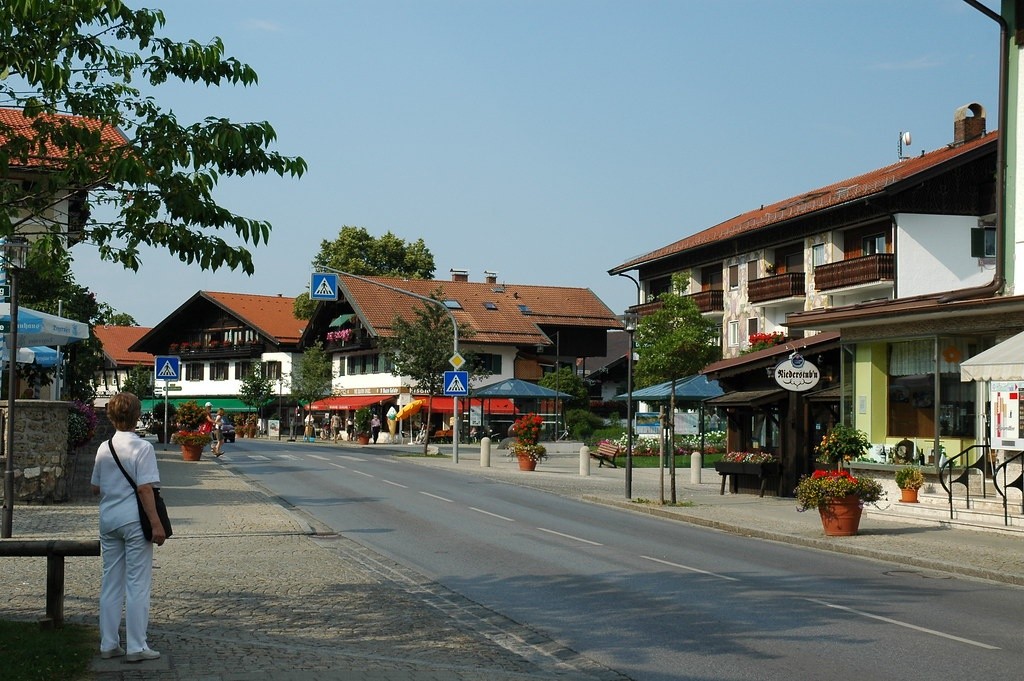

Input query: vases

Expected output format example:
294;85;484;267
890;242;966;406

818;494;863;536
718;462;781;498
194;348;201;354
209;347;219;353
517;453;536;470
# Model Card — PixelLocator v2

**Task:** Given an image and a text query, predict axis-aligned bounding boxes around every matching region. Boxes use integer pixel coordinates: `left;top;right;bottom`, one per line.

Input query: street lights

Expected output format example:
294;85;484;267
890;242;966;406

0;235;31;540
623;313;638;500
278;377;283;441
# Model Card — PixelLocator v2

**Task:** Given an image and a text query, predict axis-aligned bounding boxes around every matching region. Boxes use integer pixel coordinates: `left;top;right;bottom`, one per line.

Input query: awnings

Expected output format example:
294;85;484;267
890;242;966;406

87;398;110;407
414;396;519;414
305;396;395;410
960;331;1024;382
140;398;277;412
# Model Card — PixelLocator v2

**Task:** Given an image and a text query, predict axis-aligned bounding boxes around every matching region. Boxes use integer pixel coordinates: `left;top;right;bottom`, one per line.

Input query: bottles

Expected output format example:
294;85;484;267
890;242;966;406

938;453;945;467
919;449;924;466
880;446;886;464
888;447;893;464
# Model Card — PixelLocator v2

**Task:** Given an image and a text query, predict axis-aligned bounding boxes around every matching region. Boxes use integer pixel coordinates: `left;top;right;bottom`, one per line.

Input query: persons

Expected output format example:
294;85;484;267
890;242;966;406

372;415;380;444
203;402;225;457
92;392;166;662
323;412;354;444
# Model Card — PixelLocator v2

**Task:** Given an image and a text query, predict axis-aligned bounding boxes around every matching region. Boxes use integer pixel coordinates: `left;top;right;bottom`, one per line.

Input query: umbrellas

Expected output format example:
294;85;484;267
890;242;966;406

0;303;89;349
2;346;63;367
395;399;426;443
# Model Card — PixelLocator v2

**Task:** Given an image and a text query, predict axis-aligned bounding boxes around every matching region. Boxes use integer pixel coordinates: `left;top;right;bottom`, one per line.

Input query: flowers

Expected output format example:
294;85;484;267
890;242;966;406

171;342;201;350
738;331;784;356
74;399;97;447
815;423;872;465
506;412;546;464
720;451;776;464
210;340;219;348
327;328;353;342
793;468;889;513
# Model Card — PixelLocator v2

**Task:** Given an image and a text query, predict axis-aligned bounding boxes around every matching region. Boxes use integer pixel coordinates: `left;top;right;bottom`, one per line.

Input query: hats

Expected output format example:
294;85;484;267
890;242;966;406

205;402;213;407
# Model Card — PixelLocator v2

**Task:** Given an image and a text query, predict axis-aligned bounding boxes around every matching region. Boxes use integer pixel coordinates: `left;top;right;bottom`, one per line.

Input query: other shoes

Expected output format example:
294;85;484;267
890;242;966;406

102;646;125;659
127;646;161;663
217;450;225;458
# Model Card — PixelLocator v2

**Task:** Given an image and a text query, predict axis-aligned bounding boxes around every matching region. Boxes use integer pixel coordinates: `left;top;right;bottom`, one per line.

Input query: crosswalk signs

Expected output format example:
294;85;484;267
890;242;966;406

311;272;339;301
444;371;468;396
155;355;180;381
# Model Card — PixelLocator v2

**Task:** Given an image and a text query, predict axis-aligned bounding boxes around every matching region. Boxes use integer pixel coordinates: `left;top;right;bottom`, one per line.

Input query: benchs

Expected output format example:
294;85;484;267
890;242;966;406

429;429;453;443
590;442;619;468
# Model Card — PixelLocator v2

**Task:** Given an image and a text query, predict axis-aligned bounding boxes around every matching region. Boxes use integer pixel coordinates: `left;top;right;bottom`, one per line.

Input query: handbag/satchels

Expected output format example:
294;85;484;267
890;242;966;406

135;487;173;542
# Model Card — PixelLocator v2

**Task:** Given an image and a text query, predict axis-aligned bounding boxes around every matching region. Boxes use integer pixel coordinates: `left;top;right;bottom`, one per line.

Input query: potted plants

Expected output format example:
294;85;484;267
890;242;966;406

764;264;777;277
223;340;232;351
153;400;177;443
173;399;212;461
354;407;371;445
896;466;926;503
245;413;258;438
234;415;246;438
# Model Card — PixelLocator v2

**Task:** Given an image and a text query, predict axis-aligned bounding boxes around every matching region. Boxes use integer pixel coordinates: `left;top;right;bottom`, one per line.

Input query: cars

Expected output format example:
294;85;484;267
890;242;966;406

211;413;235;443
135;418;146;437
